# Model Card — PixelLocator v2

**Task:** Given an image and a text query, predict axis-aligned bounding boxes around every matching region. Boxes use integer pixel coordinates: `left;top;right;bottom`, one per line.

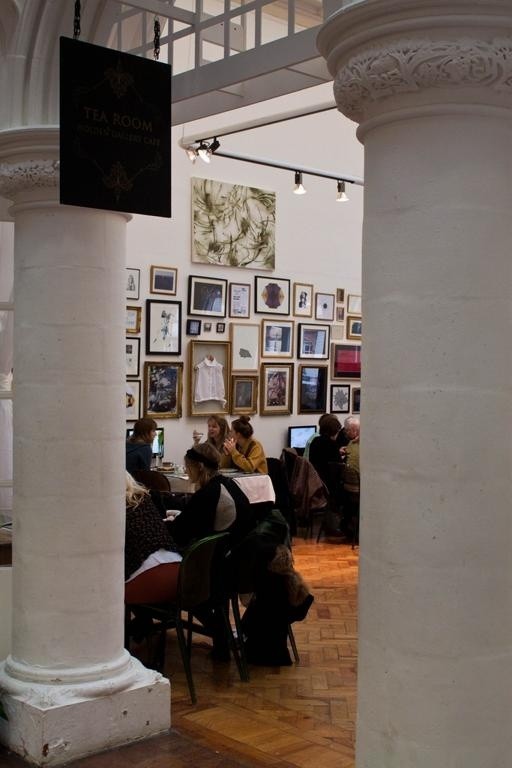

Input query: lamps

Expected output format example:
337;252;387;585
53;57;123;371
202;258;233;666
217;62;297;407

186;135;350;203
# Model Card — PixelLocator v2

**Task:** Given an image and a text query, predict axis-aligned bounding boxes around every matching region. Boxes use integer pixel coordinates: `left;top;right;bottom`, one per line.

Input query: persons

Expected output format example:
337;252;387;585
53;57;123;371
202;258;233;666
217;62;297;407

124;469;172;643
218;415;269;475
162;442;250;661
191;413;232;454
127;417;157;478
302;414;359;543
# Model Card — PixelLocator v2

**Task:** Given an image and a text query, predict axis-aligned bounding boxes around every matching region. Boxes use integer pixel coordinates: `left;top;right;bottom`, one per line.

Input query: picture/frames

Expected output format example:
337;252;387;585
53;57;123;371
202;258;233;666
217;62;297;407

126;266;362;422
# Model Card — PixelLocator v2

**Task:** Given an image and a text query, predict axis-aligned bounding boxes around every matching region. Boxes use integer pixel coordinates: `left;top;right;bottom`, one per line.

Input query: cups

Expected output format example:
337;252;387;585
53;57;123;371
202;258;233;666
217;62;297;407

194;432;203;439
174;461;185;475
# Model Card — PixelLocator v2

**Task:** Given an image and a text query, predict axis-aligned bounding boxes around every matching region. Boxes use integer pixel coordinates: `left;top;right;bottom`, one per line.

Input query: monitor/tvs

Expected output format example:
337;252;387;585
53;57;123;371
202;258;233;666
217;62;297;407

287;424;317;450
127;427;165;456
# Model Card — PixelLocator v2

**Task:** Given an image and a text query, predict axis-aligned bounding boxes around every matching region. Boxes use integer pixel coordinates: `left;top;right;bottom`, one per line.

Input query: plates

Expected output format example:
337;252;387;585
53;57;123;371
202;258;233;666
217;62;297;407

219;468;238;473
